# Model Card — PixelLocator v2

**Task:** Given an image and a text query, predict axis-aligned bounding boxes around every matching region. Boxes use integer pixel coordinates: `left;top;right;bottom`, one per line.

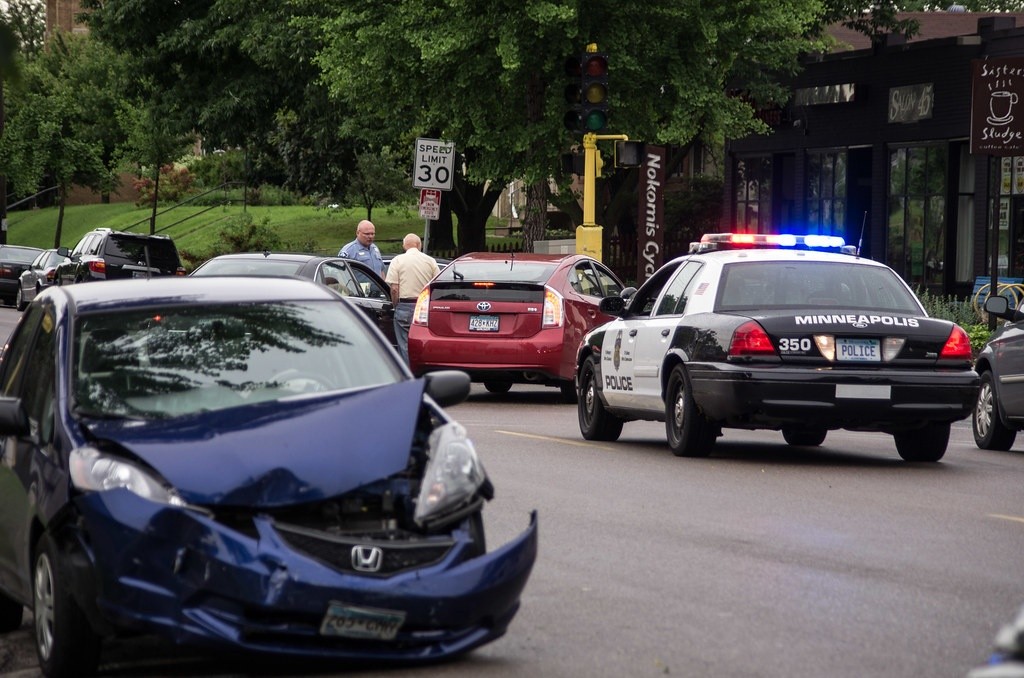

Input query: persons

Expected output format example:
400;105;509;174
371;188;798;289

338;220;385;279
386;233;441;370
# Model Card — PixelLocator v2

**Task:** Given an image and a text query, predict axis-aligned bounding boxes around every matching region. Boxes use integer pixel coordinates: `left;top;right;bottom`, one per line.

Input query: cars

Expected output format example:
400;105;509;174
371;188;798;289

0;271;539;676
972;294;1024;451
188;254;398;349
16;249;76;311
370;256;451;281
574;232;982;463
407;252;625;400
0;245;44;306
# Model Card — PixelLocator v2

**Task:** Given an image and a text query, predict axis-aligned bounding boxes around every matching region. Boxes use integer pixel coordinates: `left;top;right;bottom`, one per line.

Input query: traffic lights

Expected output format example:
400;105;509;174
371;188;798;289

615;140;644;167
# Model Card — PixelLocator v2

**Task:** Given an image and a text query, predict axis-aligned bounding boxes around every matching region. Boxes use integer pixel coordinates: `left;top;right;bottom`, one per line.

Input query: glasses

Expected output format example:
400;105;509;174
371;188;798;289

359;230;376;236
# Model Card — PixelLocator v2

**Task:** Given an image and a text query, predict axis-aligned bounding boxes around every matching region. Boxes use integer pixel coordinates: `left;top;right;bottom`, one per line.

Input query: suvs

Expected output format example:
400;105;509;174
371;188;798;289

53;227;186;287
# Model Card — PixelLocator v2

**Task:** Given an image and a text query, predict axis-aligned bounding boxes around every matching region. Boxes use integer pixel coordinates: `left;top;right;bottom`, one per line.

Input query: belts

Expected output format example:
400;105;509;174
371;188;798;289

398;298;416;303
